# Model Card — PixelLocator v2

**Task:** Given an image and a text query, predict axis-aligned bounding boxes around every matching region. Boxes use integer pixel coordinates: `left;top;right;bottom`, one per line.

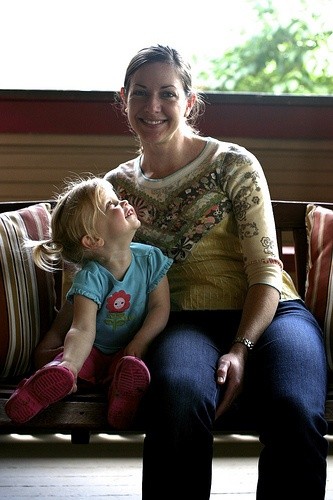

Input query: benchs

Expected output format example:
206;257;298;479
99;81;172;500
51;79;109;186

0;197;332;445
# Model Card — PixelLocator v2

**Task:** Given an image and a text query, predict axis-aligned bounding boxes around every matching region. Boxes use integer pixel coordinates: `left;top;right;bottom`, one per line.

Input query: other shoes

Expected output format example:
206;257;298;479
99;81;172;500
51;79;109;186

4;361;75;427
107;356;151;435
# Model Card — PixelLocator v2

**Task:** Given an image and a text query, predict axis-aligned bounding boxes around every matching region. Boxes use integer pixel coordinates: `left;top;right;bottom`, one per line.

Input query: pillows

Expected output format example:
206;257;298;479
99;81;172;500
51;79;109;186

0;204;61;382
301;204;332;386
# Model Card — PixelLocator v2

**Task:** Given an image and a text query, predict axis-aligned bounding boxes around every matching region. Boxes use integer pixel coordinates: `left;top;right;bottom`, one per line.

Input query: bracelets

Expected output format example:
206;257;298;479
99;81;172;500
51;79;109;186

232;337;254;353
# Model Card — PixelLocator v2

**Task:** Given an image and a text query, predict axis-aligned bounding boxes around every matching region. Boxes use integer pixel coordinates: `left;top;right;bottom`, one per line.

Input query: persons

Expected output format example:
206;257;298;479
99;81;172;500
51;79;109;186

4;177;173;429
31;45;328;500
